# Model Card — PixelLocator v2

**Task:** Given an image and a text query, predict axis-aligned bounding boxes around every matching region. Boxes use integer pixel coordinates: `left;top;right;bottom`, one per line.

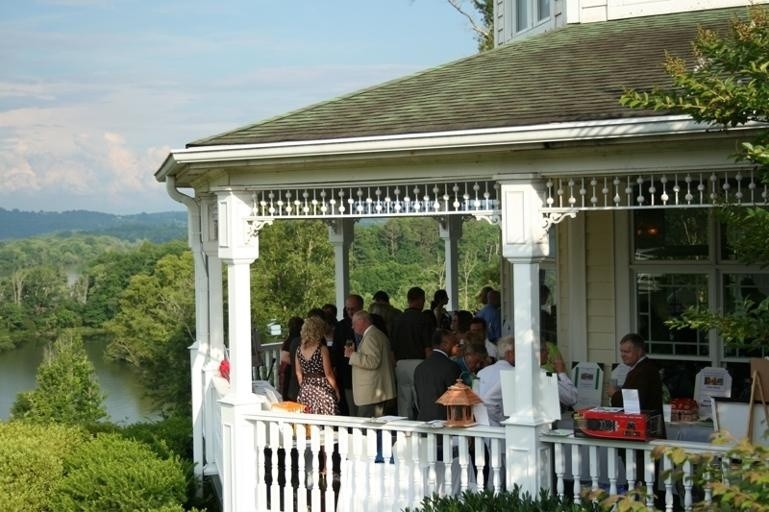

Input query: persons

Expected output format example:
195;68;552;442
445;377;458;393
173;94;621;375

279;284;515;445
607;334;666;501
540;286;578;433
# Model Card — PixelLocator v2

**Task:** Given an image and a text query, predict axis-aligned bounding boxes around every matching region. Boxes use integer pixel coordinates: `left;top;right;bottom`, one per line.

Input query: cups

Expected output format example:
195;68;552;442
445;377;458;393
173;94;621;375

345;335;352;348
